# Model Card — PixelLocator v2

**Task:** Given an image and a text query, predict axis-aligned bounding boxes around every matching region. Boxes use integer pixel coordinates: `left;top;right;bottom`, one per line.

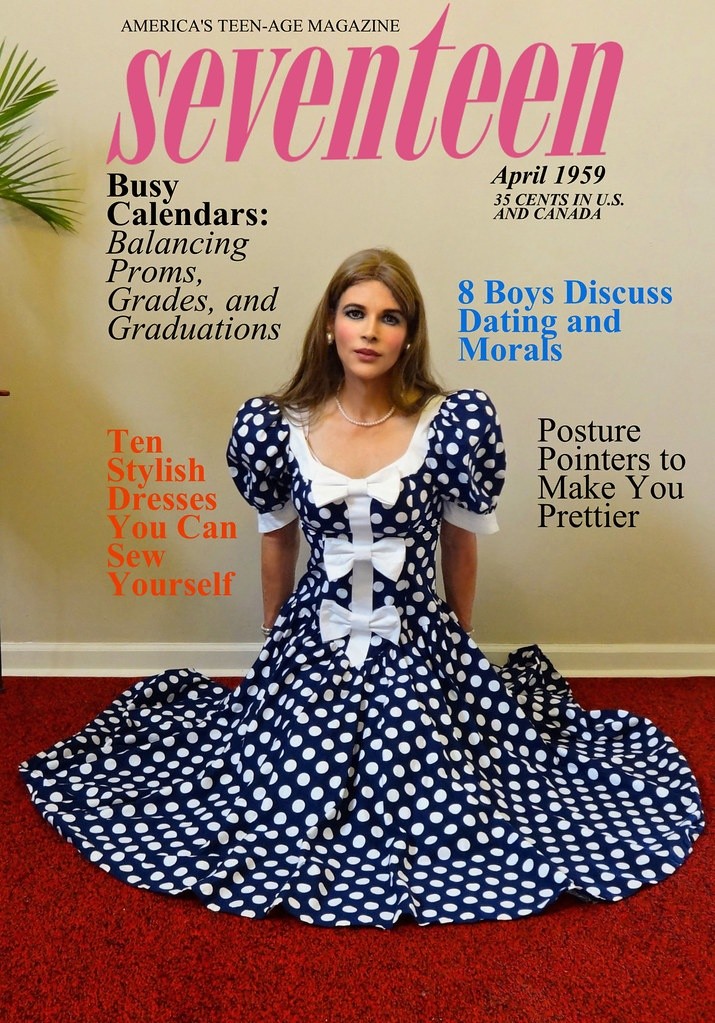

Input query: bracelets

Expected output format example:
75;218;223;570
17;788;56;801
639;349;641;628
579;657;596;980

261;624;271;635
466;629;474;637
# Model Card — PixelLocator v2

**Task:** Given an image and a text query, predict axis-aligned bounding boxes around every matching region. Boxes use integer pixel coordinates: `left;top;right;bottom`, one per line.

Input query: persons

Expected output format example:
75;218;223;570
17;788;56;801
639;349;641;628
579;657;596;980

18;248;706;930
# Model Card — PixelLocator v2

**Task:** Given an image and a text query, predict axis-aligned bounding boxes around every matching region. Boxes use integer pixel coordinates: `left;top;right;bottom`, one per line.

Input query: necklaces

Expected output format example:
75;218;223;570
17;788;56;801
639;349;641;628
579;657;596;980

334;377;396;426
